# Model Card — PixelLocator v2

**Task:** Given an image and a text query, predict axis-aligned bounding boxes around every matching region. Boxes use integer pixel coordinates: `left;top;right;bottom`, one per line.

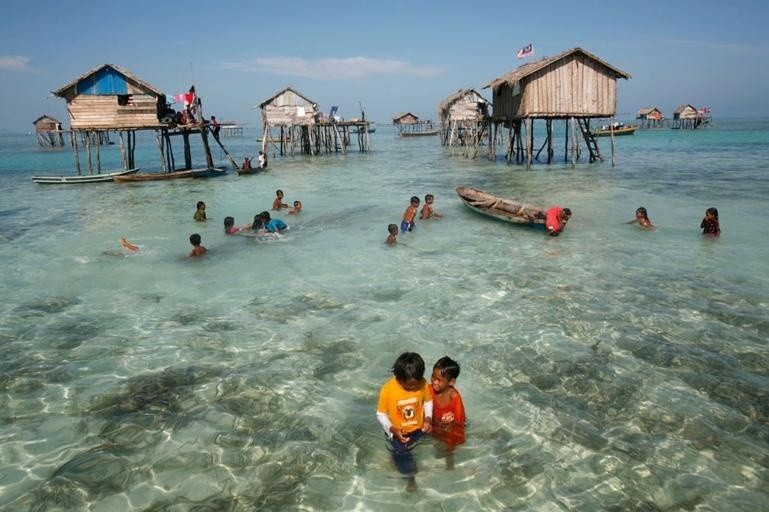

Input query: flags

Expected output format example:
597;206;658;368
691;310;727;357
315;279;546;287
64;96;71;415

517;46;533;57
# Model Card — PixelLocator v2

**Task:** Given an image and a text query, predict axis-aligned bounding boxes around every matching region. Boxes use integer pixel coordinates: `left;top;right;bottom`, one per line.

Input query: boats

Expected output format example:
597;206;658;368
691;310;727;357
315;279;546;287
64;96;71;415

236;155;267;175
108;167;225;182
456;185;572;230
256;136;297;142
108;139;115;145
584;124;640;137
402;131;438;137
30;167;141;184
349;129;376;134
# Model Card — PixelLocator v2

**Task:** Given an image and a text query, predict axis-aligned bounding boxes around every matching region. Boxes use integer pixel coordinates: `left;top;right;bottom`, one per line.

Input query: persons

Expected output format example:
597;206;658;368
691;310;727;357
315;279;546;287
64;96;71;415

423;195;443;221
385;224;399;248
429;356;465;470
179;86;267;169
601;121;624;131
545;208;568;236
375;352;433;492
525;206;571;221
625;207;651;228
120;190;301;260
401;195;420;234
700;207;721;238
